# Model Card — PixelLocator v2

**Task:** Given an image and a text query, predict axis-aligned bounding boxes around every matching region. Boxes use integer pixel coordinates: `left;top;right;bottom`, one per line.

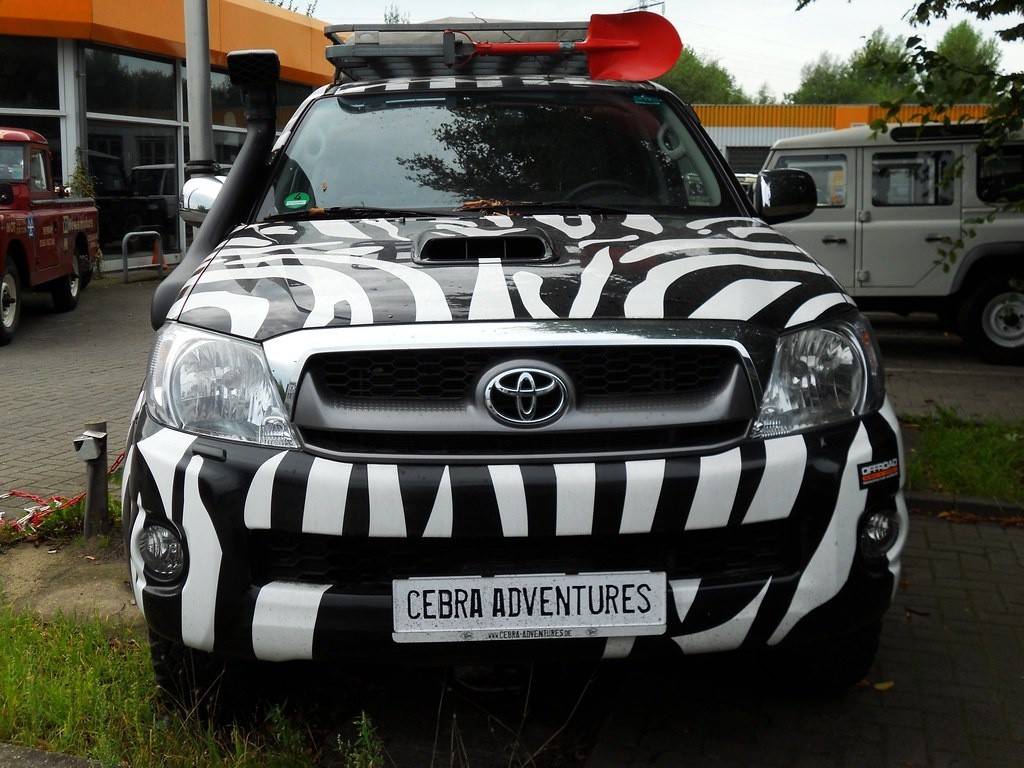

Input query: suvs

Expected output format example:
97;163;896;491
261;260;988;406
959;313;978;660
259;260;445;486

127;163;179;228
743;118;1023;368
115;13;912;734
80;150;156;251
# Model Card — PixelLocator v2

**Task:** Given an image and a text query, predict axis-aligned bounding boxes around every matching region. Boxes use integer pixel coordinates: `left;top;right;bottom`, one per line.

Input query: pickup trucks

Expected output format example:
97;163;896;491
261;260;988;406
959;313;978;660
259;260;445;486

0;127;99;346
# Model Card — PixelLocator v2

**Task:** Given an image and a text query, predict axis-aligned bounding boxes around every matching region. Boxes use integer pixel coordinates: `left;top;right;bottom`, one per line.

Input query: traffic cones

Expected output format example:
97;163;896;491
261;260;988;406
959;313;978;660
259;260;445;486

151;238;167;268
